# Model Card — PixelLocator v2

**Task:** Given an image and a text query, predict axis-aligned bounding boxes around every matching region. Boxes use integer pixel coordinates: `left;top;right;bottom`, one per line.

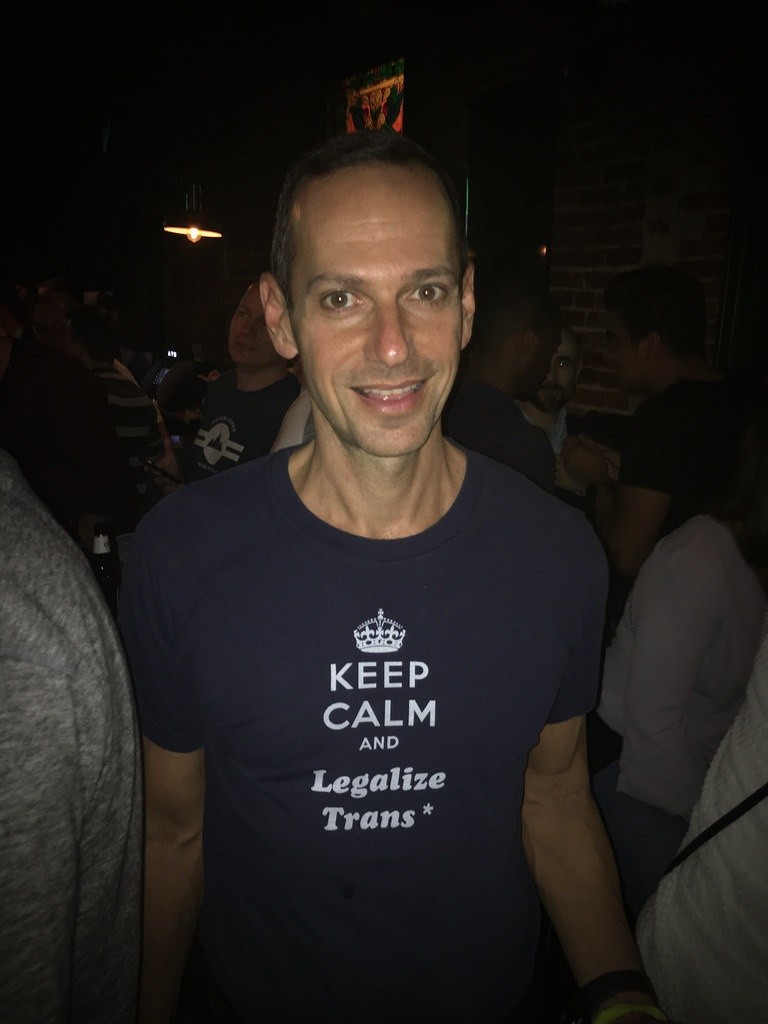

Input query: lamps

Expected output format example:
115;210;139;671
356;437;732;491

163;213;225;244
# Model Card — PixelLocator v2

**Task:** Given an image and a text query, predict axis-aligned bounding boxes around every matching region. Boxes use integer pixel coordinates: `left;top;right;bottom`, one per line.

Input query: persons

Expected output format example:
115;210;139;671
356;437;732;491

0;123;768;1024
